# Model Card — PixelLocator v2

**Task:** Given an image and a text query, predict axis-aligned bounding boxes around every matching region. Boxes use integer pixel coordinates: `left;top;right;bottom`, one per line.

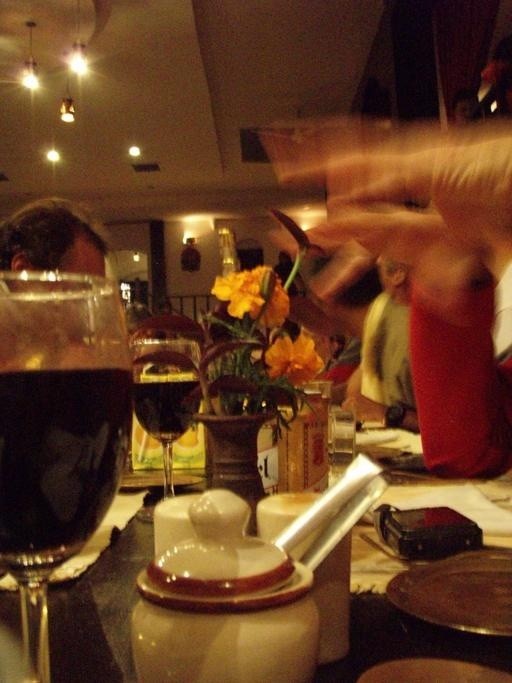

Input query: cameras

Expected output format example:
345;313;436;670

380;506;485;558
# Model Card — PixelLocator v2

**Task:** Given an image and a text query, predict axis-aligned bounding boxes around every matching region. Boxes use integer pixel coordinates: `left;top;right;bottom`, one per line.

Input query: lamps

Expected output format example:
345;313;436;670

60;48;75;123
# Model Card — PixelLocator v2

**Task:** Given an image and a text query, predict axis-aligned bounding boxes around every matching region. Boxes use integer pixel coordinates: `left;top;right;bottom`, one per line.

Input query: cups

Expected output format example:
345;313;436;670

326;402;357;488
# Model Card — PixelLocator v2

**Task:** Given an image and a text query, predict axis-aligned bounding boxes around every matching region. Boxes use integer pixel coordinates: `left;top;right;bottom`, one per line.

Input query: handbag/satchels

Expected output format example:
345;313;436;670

374;503;484;562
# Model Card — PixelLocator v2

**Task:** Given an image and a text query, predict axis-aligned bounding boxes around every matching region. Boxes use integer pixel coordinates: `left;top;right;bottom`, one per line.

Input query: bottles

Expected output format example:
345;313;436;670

127;491;321;683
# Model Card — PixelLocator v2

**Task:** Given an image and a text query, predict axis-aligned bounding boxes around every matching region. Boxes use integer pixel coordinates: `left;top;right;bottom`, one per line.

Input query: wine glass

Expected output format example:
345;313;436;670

0;273;132;683
129;338;203;500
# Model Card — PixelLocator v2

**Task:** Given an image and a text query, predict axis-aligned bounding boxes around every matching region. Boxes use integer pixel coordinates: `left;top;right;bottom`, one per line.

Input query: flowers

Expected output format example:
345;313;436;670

128;209;330;443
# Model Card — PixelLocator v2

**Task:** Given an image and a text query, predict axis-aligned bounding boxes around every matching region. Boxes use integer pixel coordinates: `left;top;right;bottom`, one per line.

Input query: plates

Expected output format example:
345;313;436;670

388;551;512;641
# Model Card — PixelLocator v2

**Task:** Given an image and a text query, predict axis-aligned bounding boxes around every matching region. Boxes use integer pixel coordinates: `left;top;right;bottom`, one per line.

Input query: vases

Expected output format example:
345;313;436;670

192;411;269;537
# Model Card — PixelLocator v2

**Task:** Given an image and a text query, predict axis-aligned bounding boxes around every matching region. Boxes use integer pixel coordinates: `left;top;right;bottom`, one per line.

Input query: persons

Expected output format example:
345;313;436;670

256;116;511;482
0;193;114;375
